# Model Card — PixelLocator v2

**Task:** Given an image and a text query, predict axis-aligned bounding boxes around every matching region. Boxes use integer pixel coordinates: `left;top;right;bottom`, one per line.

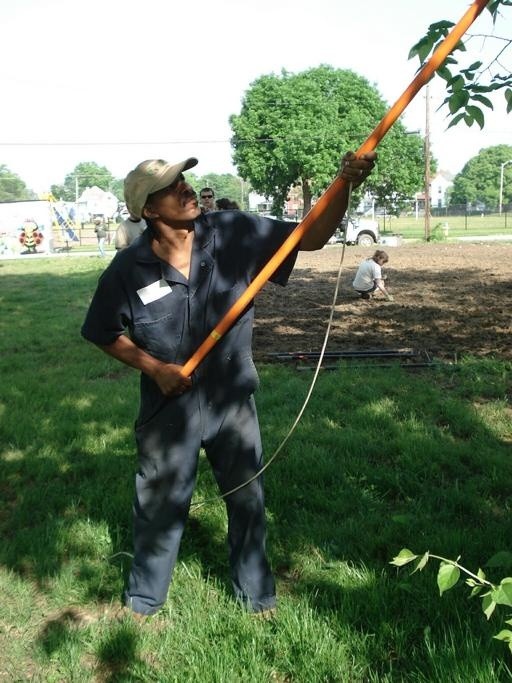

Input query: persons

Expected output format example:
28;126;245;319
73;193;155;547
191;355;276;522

353;249;394;300
80;149;377;620
93;218;107;256
114;204;148;252
215;197;240;211
199;187;217;211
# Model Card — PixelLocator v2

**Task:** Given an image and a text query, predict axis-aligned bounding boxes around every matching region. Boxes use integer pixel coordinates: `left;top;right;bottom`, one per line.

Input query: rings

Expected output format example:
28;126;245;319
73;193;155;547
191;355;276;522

359;169;363;177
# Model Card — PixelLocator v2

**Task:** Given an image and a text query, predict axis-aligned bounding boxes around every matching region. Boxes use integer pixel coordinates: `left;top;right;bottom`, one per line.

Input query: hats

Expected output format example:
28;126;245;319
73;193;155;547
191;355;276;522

124;158;198;220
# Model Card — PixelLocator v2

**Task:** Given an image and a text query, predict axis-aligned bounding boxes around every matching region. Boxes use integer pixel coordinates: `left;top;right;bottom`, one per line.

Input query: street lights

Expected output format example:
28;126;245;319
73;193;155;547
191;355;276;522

498;159;512;216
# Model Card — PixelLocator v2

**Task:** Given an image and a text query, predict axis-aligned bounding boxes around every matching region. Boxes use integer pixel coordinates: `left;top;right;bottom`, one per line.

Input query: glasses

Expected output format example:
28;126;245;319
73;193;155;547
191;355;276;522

202;195;212;198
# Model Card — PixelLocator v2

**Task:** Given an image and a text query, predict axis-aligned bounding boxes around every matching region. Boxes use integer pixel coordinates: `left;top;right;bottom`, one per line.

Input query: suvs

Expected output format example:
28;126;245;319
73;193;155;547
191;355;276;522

330;212;379;246
